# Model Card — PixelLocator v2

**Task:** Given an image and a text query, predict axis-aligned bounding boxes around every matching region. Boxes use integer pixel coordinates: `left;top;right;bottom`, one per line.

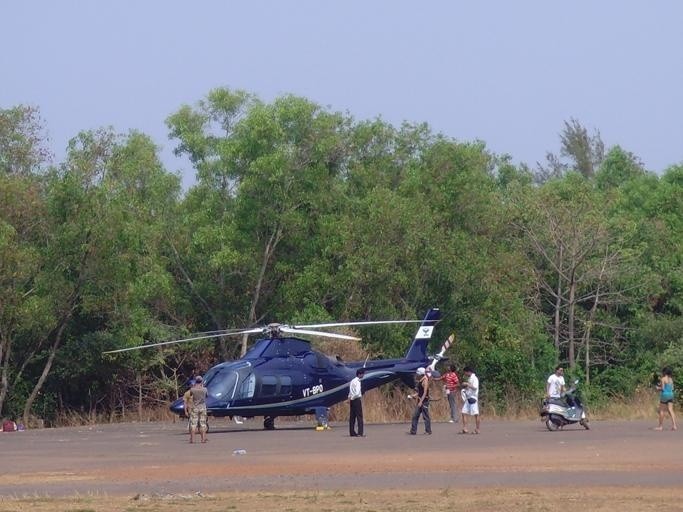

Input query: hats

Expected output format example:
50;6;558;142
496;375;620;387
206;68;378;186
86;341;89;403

189;376;203;385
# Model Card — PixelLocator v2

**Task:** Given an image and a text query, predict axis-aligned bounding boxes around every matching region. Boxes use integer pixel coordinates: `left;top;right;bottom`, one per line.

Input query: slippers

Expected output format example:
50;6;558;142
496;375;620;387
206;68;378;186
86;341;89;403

458;429;481;434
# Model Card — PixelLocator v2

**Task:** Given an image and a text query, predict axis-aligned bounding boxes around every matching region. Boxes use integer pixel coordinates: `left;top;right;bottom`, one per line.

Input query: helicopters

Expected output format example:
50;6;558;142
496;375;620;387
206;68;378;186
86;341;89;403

102;307;455;431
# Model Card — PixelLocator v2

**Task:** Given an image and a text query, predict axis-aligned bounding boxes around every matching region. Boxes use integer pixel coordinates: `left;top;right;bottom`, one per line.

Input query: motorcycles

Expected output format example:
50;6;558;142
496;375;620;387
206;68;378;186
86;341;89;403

539;380;591;430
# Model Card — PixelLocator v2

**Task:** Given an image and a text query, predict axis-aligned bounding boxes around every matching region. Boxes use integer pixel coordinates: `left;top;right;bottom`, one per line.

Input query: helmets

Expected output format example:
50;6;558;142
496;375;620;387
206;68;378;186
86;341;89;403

416;367;425;375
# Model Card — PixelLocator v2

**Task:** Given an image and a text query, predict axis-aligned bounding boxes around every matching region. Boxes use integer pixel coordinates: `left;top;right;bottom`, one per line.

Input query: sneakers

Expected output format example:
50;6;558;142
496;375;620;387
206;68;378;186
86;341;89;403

350;434;366;437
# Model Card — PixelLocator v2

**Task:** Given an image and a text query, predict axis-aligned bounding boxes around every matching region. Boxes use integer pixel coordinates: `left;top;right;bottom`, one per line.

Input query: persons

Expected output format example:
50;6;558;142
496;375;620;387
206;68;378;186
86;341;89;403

407;366;432;435
457;366;480;434
546;366;566;429
189;375;209;443
348;368;366;436
432;364;459;423
184;379;209;441
652;366;677;431
